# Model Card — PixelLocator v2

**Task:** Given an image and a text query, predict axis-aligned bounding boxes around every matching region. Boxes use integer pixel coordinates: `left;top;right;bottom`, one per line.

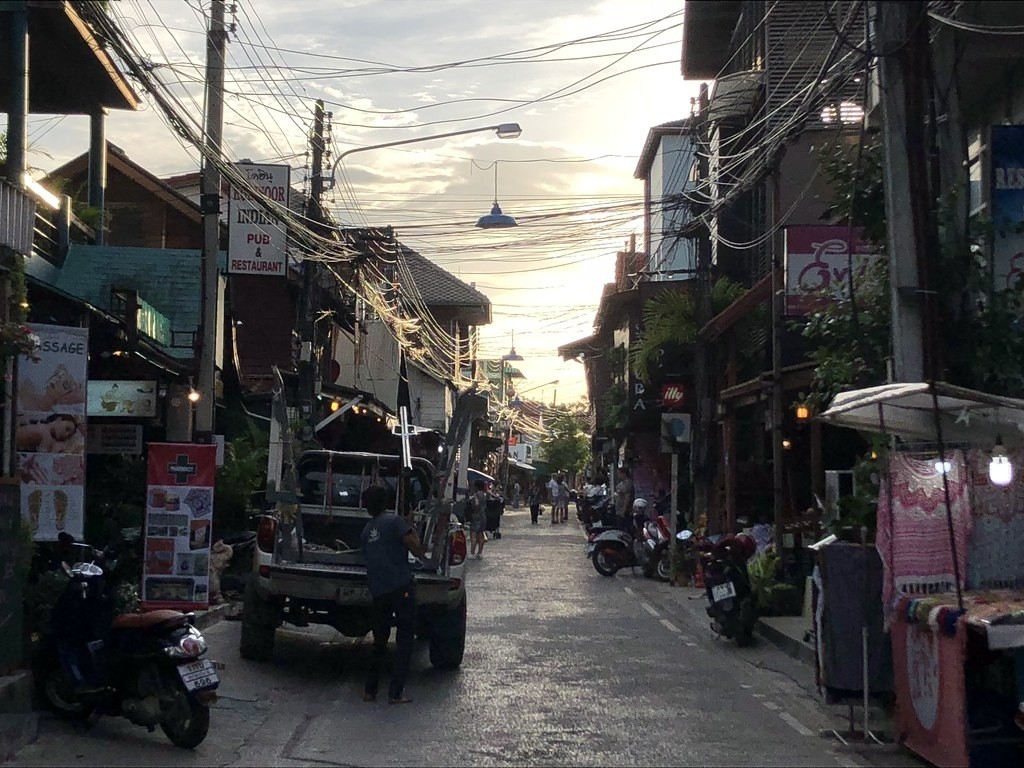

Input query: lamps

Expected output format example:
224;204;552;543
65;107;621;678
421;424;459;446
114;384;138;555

986;408;1014;486
475;163;519;228
187;379;200;402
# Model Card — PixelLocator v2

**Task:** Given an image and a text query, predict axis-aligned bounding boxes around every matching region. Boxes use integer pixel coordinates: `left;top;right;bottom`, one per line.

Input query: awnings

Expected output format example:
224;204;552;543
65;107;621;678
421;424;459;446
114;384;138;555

516;462;536;470
468;467;495;481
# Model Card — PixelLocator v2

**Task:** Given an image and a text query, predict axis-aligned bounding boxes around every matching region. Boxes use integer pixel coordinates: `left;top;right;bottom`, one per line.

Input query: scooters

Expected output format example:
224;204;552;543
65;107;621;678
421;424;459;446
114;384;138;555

584;499;680;582
676;516;758;647
569;482;621;533
30;521;225;750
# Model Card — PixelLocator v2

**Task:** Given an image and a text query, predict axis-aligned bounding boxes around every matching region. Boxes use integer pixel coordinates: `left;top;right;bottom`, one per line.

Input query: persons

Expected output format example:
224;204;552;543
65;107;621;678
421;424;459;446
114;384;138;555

360;486;423;703
527;481;541;524
513;480;520;508
467;480;487;560
17;363;86;413
15;414;76;453
547;473;572;524
614;467;634;533
585;477;594;485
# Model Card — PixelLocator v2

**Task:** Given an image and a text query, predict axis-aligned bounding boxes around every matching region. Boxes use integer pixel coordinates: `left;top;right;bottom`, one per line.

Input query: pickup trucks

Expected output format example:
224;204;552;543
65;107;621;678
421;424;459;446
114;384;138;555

238;453;469;675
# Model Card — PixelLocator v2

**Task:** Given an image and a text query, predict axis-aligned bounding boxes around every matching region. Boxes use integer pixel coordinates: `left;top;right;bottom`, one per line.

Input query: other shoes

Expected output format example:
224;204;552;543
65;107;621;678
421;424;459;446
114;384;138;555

467;554;476;560
476;552;484;560
387;698;413;705
362;689;376;702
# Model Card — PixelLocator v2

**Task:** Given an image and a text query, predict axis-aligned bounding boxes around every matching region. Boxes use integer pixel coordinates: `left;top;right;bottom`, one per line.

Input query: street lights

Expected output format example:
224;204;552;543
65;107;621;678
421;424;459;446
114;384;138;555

298;121;527;454
500;376;563;497
